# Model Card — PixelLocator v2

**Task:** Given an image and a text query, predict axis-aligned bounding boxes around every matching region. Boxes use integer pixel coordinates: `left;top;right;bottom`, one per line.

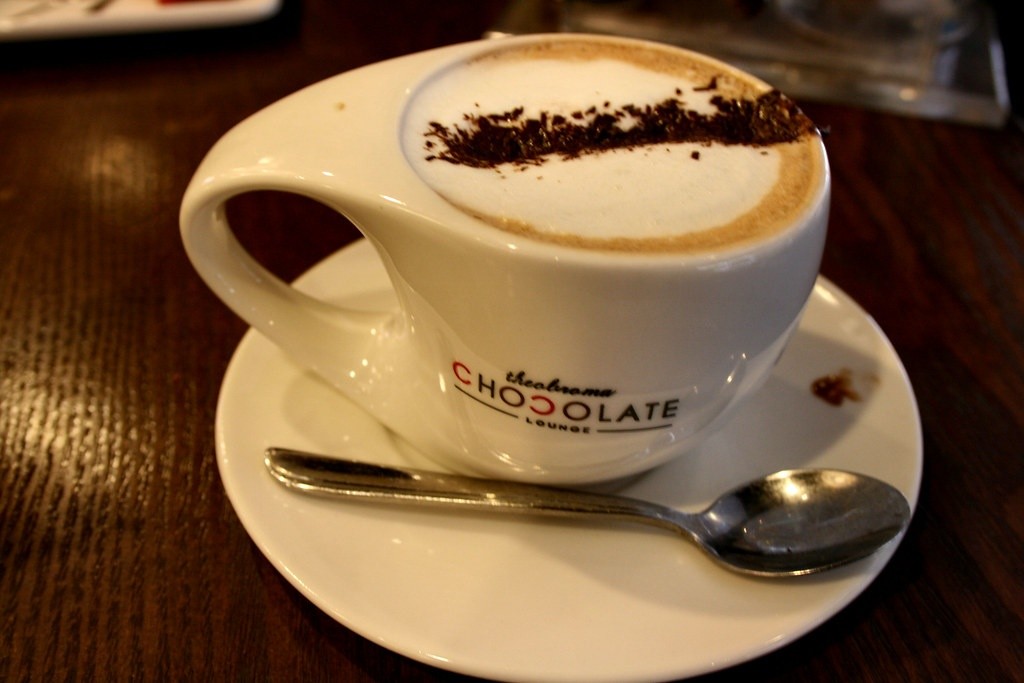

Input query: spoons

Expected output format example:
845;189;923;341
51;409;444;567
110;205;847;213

263;447;912;579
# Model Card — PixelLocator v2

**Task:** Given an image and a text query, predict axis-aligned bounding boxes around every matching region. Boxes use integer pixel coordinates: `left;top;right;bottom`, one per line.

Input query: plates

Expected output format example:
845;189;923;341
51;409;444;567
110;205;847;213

0;0;281;38
215;237;924;683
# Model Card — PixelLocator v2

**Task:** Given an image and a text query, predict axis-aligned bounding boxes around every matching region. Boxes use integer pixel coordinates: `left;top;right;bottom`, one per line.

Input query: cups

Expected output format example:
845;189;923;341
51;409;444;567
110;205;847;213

180;32;832;483
